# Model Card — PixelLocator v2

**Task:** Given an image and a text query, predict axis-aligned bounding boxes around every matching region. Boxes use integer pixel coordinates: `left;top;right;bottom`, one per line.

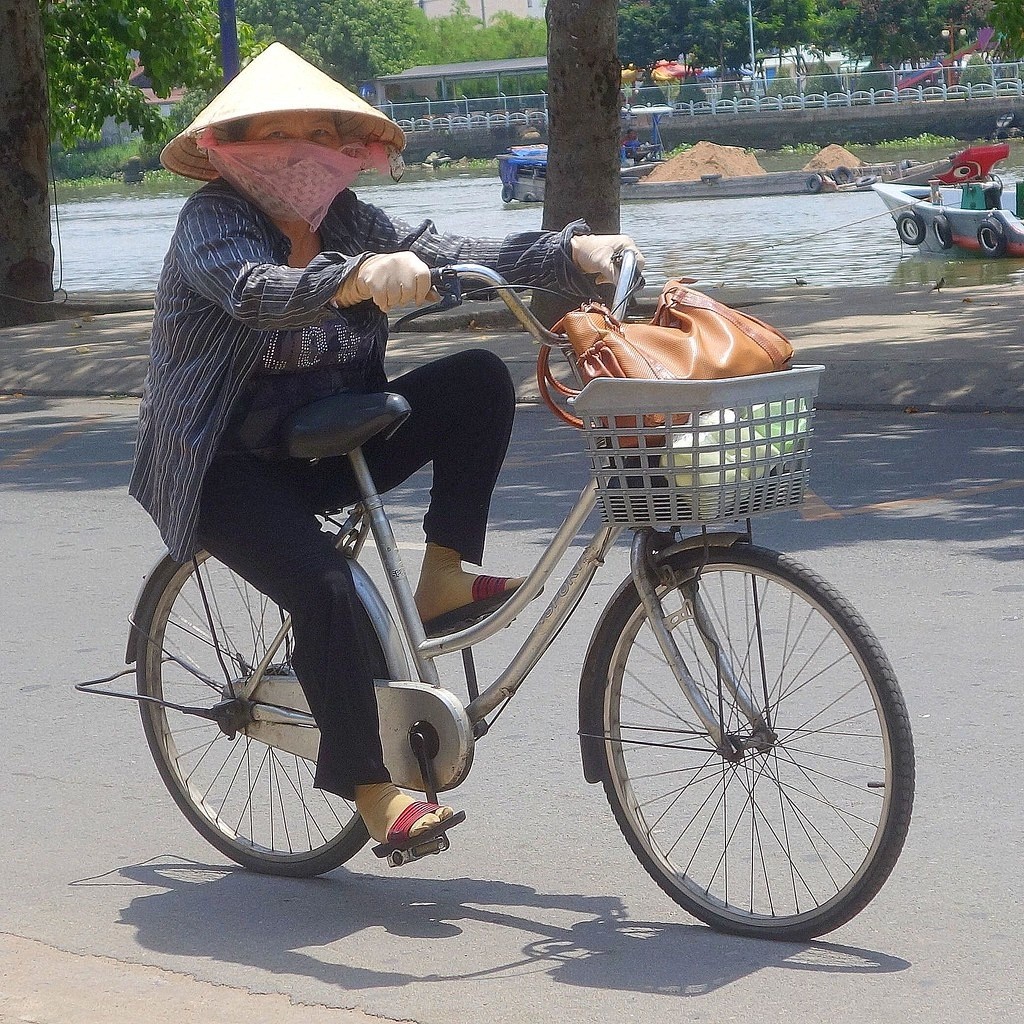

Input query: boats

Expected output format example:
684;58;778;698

871;142;1024;258
495;110;669;203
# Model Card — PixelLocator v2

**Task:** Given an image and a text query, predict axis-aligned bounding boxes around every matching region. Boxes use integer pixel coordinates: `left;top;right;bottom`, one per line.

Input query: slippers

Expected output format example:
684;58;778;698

422;574;544;636
371;800;466;859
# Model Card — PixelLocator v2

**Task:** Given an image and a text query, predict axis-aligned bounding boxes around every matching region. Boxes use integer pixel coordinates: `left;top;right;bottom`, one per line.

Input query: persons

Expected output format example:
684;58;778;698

129;42;642;861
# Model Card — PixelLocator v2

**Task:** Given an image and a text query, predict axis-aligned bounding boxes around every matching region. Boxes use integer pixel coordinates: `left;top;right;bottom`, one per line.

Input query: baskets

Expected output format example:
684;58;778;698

567;360;826;524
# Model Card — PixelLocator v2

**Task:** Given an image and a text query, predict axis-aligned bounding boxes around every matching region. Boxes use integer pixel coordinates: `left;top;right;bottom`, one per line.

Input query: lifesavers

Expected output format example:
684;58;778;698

856;174;877;187
932;215;953;250
976;217;1006;257
896;211;927;245
523;192;536;202
806;173;822;193
501;183;514;203
833;165;853;184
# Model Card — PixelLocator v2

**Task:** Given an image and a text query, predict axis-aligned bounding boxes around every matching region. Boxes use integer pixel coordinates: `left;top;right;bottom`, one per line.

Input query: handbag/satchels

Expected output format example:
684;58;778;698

536;279;794;450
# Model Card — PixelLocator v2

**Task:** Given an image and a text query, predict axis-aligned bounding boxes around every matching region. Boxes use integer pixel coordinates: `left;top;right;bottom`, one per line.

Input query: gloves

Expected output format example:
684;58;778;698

570;233;646;286
334;251;440;313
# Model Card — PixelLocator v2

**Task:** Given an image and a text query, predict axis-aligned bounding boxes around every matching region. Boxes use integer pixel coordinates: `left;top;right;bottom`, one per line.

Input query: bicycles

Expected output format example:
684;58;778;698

75;242;917;943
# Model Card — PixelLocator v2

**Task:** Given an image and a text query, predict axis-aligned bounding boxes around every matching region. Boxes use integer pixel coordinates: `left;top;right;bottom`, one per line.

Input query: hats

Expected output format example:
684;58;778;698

159;43;407;180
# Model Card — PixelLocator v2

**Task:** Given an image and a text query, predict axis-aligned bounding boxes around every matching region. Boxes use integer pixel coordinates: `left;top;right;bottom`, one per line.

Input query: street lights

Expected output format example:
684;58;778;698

942;17;967;92
